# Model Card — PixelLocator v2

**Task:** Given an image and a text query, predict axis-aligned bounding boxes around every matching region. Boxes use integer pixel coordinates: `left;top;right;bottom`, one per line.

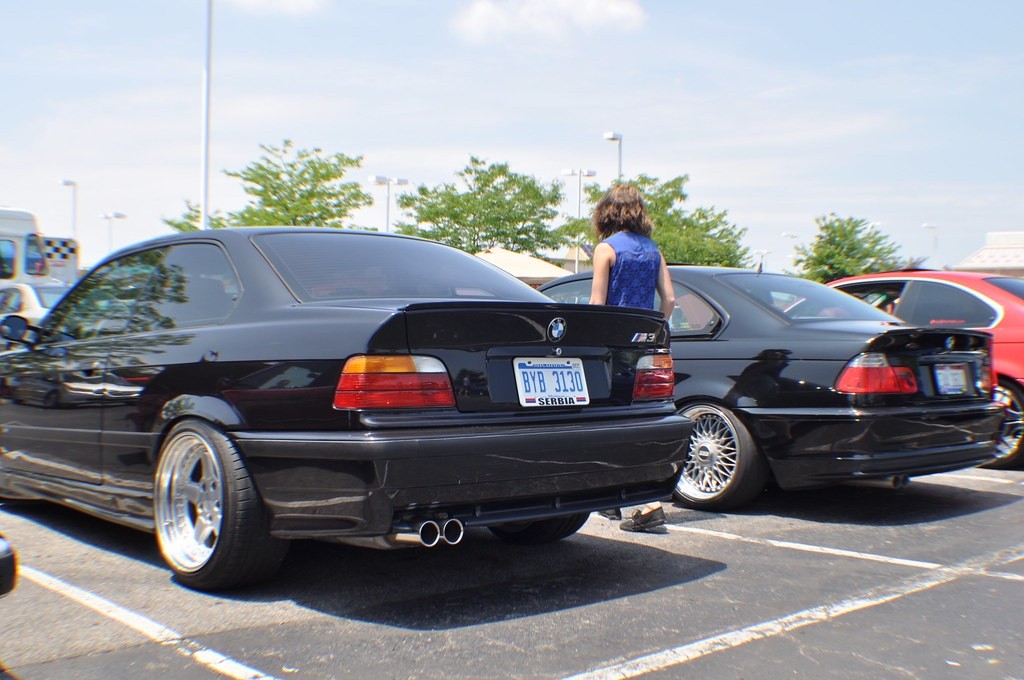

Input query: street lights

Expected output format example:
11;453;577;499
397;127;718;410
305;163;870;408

566;170;596;274
604;131;623;180
923;223;938;268
61;178;78;237
371;174;410;232
101;212;127;254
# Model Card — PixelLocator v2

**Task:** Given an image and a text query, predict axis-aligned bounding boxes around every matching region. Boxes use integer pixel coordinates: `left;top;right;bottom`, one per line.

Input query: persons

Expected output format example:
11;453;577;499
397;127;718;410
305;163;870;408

588;184;675;531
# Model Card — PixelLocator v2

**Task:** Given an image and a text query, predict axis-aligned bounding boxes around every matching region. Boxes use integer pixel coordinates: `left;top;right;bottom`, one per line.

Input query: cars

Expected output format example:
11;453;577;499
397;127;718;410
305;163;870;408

532;266;1008;513
1;226;696;594
0;279;75;349
779;270;1024;470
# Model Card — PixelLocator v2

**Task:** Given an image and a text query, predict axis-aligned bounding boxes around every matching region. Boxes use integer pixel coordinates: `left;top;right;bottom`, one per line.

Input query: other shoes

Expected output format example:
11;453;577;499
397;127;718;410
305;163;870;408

597;509;621;520
620;507;666;530
